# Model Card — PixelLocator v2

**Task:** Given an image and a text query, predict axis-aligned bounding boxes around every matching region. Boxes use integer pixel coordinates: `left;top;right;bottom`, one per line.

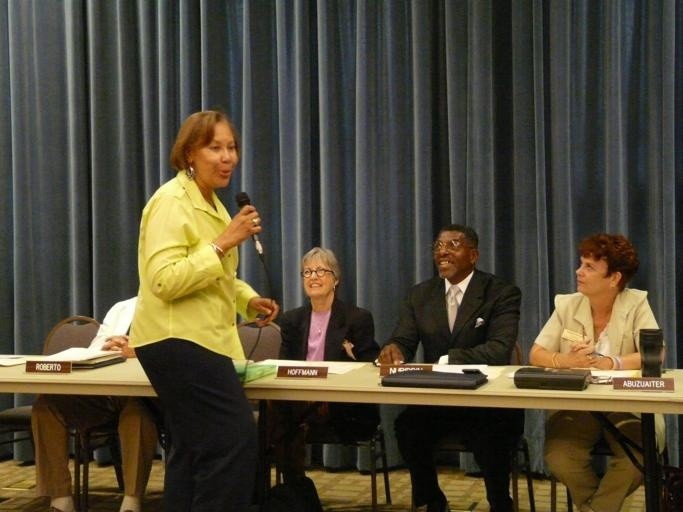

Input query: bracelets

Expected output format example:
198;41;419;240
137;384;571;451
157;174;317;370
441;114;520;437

554;351;562;370
601;354;617;370
551;350;558;369
208;240;226;257
612;356;623;369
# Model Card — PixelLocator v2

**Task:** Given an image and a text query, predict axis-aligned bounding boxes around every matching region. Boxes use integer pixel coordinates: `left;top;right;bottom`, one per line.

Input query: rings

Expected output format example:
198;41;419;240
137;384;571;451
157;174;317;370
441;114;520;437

252;217;259;226
587;355;592;360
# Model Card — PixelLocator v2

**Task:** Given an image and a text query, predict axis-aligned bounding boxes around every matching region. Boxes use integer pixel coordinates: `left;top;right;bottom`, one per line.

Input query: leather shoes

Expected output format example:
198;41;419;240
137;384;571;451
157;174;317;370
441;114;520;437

426;493;446;511
490;496;514;512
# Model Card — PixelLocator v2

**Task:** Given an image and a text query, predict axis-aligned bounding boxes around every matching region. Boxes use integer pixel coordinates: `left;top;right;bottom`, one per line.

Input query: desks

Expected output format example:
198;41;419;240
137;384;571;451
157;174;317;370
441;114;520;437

243;355;683;512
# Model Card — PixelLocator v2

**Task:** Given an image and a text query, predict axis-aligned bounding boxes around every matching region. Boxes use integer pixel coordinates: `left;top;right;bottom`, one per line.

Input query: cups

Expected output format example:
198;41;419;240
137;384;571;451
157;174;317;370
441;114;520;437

638;327;664;380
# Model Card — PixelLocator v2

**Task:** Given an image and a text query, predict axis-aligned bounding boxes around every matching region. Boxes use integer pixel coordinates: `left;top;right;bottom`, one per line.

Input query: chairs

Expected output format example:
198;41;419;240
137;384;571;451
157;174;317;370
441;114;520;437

0;315;669;512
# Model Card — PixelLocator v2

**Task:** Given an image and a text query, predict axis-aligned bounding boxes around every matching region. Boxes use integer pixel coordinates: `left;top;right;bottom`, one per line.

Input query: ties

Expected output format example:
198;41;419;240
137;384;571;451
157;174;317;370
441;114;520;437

447;286;460;334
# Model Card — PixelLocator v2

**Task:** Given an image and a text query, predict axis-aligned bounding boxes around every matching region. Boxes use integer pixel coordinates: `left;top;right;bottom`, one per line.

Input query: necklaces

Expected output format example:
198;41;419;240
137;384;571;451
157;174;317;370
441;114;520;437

312;309;329;335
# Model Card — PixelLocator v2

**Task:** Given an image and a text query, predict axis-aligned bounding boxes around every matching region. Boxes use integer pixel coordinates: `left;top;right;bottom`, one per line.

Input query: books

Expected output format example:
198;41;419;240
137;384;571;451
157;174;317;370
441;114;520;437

42;346;125;370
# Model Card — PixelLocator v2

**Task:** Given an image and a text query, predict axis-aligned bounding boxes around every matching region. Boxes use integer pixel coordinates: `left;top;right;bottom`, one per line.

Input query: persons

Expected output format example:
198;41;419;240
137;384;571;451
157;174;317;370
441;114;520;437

126;107;281;511
377;222;523;511
255;246;382;511
527;233;667;512
29;294;159;512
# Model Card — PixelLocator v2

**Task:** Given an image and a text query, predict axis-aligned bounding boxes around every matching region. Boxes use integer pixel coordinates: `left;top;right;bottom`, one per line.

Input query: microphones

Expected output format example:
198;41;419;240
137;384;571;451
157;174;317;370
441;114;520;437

234;188;266;259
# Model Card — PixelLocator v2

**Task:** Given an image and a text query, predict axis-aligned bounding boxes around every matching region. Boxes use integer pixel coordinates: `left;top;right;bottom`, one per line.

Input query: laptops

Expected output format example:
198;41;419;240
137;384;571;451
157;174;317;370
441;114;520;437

381;369;489;389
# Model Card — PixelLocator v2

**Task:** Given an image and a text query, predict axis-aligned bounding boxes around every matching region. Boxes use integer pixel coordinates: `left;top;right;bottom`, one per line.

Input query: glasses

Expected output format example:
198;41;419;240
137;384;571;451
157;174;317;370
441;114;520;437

432;239;472;251
301;268;335;278
